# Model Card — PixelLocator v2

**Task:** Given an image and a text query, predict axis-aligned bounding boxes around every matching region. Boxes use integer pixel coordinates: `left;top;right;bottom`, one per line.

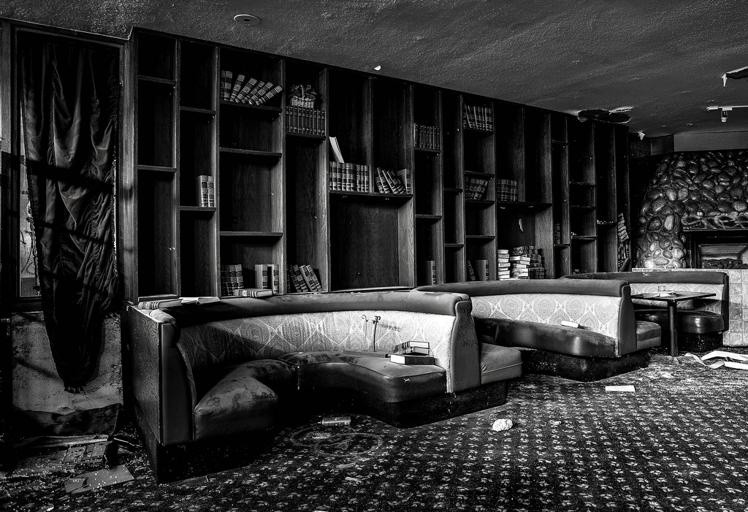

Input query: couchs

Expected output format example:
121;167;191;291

120;270;728;483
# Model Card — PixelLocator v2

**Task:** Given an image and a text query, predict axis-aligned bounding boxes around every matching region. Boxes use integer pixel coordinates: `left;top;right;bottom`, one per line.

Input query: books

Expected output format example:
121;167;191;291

413;121;441;150
288;262;322;293
220;70;283;106
463;102;494;132
466;259;489;281
496;179;519;202
554;222;562;246
424;259;436;285
498;245;546;280
390;341;435;365
198;175;214;208
372;166;411;196
137;294;220;310
222;263;279;299
604;384;636;394
285;105;326;137
561;320;582;328
464;176;489;201
329;162;370;193
329;136;345;163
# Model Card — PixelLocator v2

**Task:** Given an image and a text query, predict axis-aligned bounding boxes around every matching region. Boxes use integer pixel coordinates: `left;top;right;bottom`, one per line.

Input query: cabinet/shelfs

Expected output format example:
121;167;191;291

123;26;632;301
